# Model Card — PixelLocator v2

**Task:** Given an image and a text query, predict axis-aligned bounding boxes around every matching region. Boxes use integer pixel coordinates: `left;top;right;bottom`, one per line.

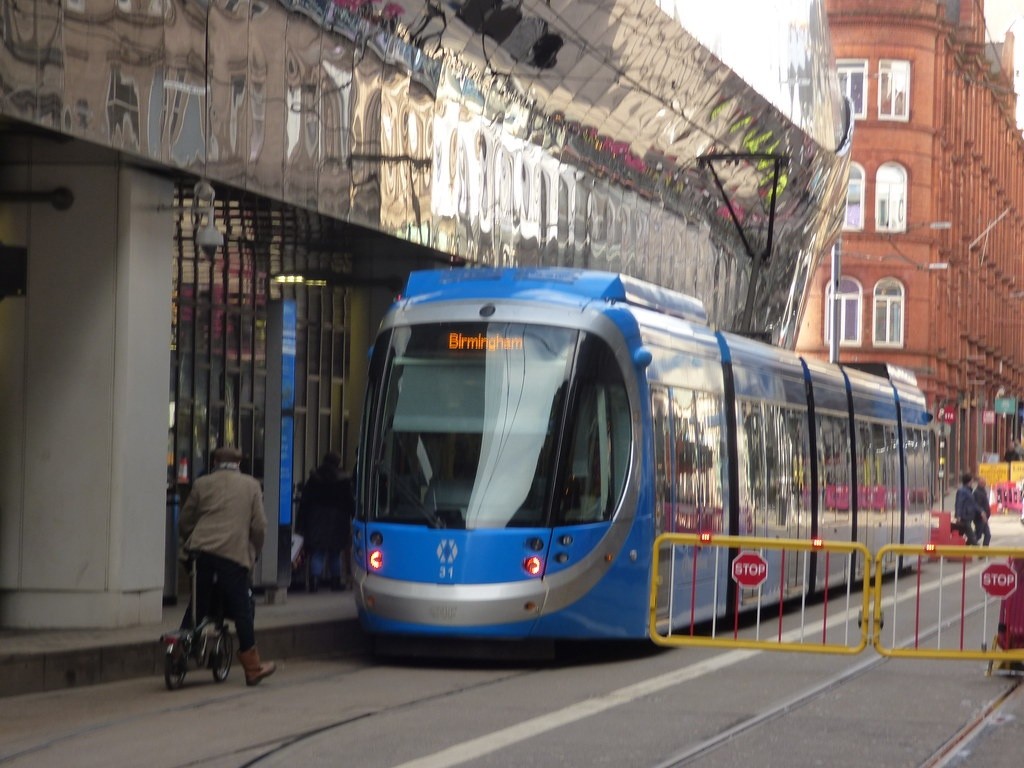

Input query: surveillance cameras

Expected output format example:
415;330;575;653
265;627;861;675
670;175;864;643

197;227;225;261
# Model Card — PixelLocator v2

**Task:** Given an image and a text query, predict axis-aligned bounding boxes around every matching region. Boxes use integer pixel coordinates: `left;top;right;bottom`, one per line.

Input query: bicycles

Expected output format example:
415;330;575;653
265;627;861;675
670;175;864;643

159;557;259;689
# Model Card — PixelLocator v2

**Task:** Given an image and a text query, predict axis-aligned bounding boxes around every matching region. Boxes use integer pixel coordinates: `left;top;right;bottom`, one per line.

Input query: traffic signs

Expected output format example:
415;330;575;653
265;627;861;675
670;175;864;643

979;562;1017;600
732;550;769;590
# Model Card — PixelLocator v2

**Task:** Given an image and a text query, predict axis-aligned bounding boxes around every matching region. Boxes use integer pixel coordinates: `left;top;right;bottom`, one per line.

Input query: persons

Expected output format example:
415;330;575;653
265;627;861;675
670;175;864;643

177;446;277;689
965;477;992;560
954;474;988;559
295;450;357;594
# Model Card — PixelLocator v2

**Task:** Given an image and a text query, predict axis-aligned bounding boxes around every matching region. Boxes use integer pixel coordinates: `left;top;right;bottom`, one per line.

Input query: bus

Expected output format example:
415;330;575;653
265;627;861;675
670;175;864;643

349;266;939;666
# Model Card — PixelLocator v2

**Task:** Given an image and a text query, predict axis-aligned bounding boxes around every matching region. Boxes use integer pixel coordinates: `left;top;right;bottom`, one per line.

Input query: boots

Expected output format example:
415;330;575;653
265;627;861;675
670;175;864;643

235;641;276;687
